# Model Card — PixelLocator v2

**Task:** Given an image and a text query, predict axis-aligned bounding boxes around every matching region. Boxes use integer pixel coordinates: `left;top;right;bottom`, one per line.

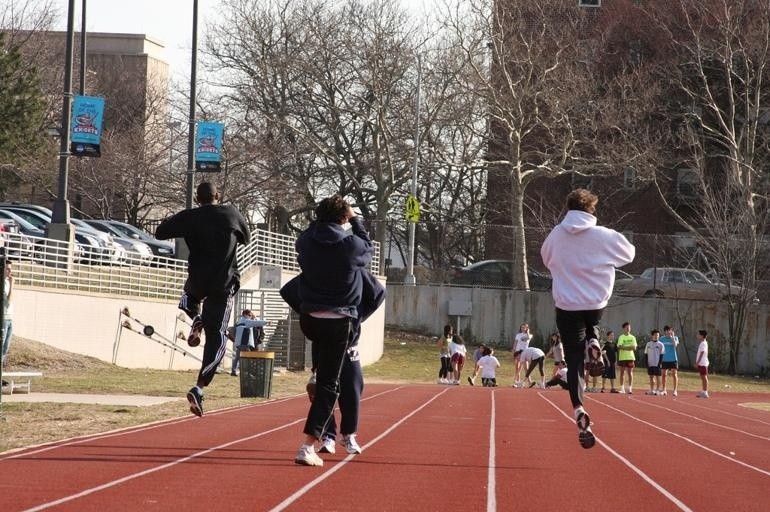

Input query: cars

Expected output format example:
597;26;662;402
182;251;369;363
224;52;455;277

614;267;759;305
0;203;174;268
448;259;552;290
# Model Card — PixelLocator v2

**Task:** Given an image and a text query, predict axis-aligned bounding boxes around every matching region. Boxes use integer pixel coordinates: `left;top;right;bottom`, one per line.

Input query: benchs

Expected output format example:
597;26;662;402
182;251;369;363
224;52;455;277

2;372;42;395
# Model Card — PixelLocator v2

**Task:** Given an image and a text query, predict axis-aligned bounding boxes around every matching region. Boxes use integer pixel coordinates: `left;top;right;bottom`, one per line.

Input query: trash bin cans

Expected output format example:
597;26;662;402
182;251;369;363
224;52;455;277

239;350;275;399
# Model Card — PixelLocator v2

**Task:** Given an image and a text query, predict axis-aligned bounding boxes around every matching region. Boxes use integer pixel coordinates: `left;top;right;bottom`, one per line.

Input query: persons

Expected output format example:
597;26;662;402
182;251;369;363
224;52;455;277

431;322;678;397
316;325;365;454
541;188;640;450
278;196;388;470
155;182;254;420
694;330;710;399
2;260;15;385
251;312;265;349
231;310;273;377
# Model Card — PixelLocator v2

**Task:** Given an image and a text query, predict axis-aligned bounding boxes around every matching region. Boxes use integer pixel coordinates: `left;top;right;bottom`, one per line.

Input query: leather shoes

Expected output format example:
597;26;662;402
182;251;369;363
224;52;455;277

231;372;238;376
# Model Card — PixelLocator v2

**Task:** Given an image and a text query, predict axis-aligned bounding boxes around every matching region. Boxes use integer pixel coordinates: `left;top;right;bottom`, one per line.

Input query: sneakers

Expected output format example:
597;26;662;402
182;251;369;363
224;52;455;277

188;315;204;347
295;444;324;467
316;436;336;454
186;387;204;416
339;433;362;454
587;340;607;377
574;405;595;449
437;377;709;398
307;372;316;403
